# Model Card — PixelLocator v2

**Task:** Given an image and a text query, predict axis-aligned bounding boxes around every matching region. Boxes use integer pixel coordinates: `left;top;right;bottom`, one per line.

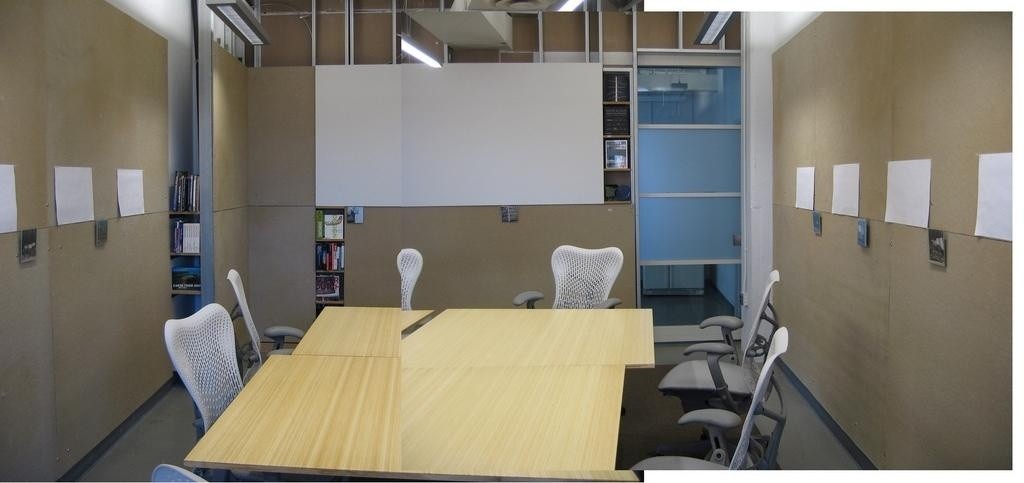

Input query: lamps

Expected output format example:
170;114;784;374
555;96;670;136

695;11;737;45
399;13;444;67
206;0;272;48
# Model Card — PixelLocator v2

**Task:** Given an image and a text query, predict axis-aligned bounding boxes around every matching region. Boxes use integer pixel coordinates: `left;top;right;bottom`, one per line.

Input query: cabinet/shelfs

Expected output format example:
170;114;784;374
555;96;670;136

168;209;201;295
603;66;632;203
315;205;347;305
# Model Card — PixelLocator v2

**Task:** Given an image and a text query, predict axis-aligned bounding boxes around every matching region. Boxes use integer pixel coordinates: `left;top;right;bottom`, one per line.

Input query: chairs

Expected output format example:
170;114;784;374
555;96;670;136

625;325;795;469
226;268;306;384
151;462;211;482
511;243;628;418
657;267;783;454
163;302;282;482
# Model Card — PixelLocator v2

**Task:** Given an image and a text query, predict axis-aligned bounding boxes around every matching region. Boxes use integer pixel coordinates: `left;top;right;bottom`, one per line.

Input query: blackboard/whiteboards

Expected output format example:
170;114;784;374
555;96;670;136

315;62;605;209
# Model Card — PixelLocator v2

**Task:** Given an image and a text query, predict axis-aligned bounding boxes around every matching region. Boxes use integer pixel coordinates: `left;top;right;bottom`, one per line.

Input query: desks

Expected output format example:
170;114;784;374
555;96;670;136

180;352;626;480
290;304;656;369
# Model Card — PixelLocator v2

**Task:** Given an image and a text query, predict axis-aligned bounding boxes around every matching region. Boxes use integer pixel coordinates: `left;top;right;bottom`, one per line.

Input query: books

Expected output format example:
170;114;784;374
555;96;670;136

173;170;200;253
315;210;345;271
